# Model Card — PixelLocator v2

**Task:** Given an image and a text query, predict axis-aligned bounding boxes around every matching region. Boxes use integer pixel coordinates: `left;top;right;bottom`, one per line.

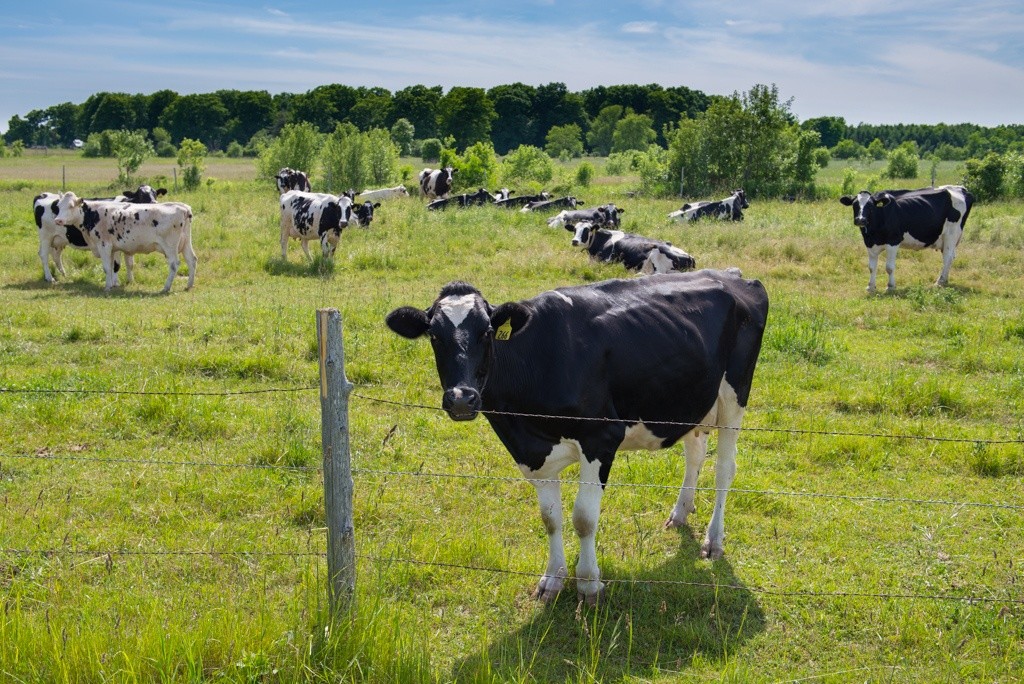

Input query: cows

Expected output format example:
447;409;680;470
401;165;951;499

427;188;581;215
360;184;409;199
55;191;197;295
565;223;696;273
33;185;167;285
547;202;626;231
278;167;311;195
385;273;769;606
279;190;381;263
839;185;974;297
419;167;458;202
666;187;751;222
